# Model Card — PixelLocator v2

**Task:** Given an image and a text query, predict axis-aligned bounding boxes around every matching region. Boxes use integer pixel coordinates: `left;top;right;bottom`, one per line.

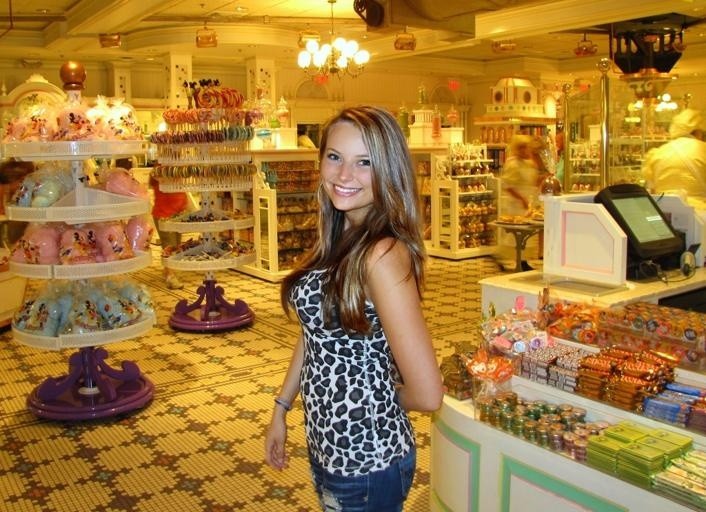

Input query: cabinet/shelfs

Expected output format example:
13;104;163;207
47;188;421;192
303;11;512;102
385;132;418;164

1;60;705;512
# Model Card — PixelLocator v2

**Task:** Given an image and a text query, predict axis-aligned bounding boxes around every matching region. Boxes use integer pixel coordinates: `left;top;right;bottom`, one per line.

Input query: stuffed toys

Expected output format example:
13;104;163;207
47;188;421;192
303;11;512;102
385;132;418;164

0;106;154;336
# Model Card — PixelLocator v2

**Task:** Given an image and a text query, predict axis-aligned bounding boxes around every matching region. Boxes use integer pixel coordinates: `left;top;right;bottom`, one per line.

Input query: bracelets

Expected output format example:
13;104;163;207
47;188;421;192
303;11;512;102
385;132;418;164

273;396;290;412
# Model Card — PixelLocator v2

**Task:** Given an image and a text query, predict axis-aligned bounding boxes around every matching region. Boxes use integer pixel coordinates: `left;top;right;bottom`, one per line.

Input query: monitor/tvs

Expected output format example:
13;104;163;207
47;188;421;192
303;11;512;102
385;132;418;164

593;184;682;281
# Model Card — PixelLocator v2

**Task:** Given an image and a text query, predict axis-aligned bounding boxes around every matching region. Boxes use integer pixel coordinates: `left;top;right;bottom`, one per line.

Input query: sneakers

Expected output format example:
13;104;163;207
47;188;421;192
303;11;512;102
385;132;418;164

521;260;535;271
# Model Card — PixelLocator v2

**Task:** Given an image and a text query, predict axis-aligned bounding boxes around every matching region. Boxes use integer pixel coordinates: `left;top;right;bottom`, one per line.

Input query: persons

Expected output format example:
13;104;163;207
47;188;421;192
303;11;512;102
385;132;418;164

495;133;539;273
555;131;566;186
262;105;448;512
532;136;554;261
640;106;706;207
148;150;195;291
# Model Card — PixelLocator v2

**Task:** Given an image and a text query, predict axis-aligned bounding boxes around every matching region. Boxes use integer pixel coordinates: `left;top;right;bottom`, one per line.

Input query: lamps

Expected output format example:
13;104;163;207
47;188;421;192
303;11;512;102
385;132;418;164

298;25;368;80
394;32;418;51
97;31;122;49
195;25;218;48
575;32;599;56
299;29;321;46
492;40;517;55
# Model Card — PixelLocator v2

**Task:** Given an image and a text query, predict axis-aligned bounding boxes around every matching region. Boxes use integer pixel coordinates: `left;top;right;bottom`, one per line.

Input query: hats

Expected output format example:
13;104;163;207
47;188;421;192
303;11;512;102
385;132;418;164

670;108;705;138
510;134;532;150
